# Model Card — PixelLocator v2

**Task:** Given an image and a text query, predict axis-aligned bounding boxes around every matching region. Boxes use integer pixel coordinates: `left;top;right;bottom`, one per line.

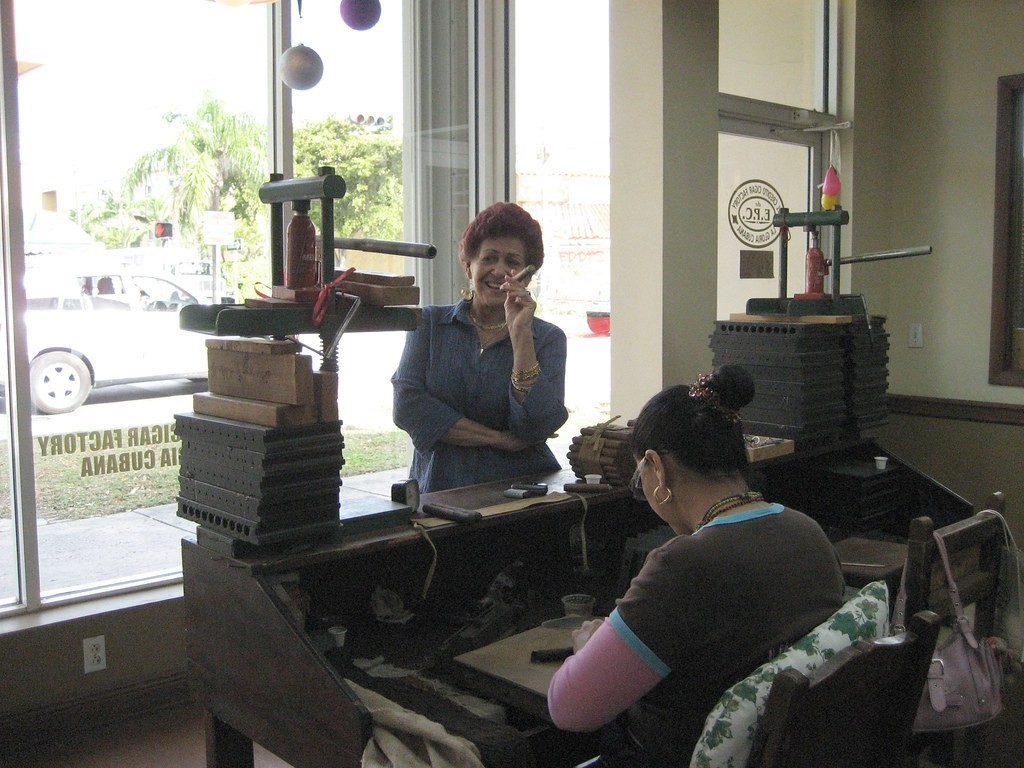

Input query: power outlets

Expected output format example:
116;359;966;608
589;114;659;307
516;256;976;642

82;634;106;674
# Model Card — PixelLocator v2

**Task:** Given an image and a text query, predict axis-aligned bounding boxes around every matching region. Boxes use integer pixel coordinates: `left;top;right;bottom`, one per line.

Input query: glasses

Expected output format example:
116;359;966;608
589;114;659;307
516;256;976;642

629;449;669;501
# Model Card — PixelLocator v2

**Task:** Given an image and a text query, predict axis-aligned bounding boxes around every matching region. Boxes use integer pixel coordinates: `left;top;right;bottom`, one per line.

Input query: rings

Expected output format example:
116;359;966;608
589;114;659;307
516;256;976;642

527;291;531;296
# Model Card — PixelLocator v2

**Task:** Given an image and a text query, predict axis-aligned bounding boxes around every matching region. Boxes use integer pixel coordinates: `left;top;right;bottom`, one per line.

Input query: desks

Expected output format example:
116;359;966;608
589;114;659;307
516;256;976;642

180;429;976;768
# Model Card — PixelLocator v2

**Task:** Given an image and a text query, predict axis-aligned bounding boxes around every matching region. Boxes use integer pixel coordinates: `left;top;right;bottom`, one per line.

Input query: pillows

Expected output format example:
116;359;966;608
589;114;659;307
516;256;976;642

690;580;891;768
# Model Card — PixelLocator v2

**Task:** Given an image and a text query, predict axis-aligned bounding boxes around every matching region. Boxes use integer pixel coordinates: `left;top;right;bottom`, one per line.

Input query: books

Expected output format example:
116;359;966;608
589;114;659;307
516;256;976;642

743;433;794;462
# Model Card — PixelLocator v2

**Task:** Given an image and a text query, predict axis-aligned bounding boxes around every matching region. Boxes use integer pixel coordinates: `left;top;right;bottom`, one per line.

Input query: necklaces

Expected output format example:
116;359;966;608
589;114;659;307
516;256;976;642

696;491;764;530
469;309;507;347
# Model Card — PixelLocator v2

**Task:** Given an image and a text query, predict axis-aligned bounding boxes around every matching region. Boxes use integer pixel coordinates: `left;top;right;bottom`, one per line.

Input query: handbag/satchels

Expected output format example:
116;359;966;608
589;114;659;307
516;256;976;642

977;507;1024;673
885;531;1003;734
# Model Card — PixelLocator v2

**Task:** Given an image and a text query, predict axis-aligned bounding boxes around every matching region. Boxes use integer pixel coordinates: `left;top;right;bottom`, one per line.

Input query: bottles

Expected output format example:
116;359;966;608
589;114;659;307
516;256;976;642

287;199;315;290
805;231;824;294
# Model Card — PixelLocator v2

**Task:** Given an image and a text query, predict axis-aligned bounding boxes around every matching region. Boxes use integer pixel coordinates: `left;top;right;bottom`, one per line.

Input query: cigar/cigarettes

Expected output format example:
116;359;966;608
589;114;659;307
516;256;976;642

566;418;636;486
530;645;573;662
564;483;612;491
500;265;535;292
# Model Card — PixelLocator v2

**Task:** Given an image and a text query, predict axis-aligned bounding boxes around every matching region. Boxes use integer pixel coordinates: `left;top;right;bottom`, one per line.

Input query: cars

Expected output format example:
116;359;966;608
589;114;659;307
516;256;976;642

0;241;237;416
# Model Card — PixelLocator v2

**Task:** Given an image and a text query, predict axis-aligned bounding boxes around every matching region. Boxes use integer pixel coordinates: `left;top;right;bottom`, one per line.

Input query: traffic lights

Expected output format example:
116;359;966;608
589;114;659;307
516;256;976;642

155;222;173;238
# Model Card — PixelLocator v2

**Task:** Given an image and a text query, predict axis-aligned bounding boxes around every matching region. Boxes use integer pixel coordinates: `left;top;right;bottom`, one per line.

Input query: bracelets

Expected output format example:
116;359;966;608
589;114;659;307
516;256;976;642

511;361;542;391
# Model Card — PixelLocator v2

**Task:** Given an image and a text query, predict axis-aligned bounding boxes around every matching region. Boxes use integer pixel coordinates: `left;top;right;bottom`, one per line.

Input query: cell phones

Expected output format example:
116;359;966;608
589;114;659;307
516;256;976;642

510;482;548;495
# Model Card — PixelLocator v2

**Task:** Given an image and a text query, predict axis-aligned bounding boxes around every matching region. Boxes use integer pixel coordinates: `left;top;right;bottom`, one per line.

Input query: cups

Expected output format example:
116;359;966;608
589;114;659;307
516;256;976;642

562;594;595;633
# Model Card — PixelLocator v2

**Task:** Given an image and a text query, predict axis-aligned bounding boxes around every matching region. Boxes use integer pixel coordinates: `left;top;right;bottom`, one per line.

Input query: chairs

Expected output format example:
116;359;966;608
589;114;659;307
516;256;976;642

894;490;1007;768
751;608;941;768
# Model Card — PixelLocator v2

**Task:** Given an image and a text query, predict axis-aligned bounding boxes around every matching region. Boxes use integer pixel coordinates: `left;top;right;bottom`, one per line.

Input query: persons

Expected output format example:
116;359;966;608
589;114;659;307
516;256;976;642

98;277;115;294
390;201;568;493
549;364;846;768
